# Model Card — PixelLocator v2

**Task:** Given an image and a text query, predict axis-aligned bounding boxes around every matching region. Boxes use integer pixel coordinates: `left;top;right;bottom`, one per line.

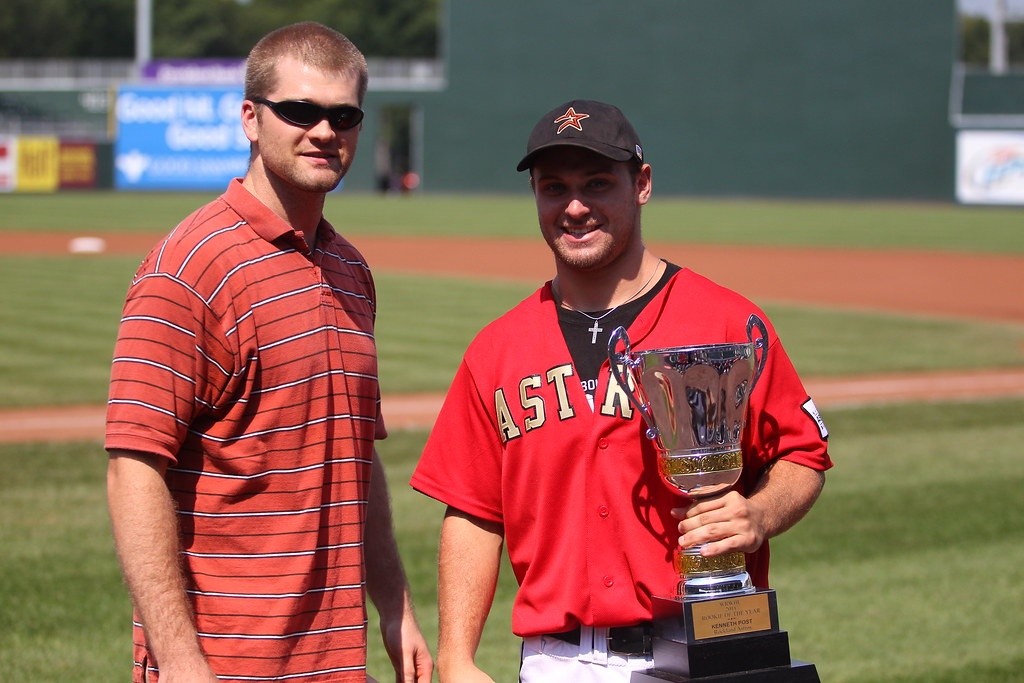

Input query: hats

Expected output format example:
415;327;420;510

516;100;642;172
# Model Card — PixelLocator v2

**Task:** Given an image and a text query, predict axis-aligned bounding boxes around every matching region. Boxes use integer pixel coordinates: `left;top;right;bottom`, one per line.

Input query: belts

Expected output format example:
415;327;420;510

543;624;654;655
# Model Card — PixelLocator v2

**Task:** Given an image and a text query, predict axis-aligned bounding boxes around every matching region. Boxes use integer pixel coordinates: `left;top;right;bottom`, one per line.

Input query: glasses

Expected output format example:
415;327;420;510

248;95;364;130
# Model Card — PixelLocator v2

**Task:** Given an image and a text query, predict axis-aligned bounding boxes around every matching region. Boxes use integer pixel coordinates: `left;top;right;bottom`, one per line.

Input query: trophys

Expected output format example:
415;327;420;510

604;312;824;683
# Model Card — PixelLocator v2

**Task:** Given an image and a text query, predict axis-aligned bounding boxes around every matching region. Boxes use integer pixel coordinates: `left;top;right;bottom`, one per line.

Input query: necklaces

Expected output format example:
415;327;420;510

545;255;662;347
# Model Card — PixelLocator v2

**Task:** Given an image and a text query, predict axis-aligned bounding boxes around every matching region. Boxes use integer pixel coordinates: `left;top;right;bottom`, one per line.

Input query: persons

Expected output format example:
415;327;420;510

404;96;836;683
98;16;436;683
